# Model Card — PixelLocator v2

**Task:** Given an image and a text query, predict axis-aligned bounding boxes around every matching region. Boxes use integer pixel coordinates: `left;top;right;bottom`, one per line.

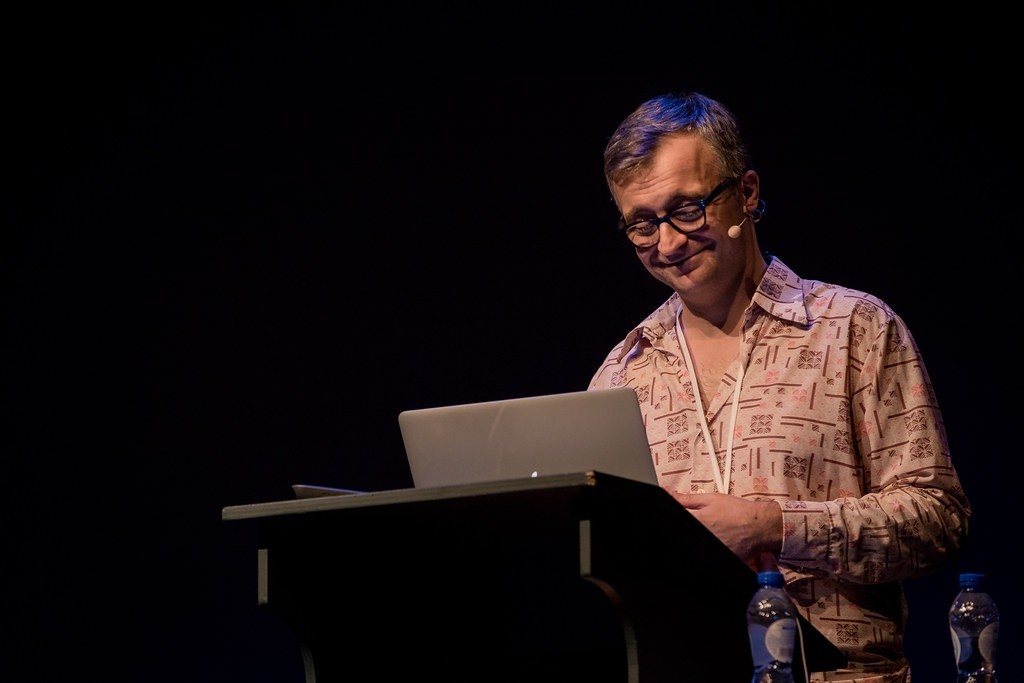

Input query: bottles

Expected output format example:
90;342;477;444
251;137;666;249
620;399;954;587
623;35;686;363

747;571;796;683
948;573;999;683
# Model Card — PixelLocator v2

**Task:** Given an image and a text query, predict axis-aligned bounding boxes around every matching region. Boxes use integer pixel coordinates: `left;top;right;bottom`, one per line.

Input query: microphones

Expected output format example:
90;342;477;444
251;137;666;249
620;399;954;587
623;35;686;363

728;216;747;240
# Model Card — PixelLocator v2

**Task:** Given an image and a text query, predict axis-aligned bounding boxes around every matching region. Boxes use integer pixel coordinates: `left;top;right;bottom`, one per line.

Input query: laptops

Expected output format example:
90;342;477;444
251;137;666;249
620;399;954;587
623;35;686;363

399;387;659;490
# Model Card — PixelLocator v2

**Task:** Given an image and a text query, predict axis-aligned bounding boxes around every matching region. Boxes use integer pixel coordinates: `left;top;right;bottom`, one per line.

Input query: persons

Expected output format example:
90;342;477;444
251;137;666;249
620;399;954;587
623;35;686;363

584;94;972;683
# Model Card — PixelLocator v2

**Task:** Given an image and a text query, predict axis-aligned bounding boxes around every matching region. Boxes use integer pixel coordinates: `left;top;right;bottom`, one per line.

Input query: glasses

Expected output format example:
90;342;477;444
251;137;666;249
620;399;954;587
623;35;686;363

616;174;737;250
